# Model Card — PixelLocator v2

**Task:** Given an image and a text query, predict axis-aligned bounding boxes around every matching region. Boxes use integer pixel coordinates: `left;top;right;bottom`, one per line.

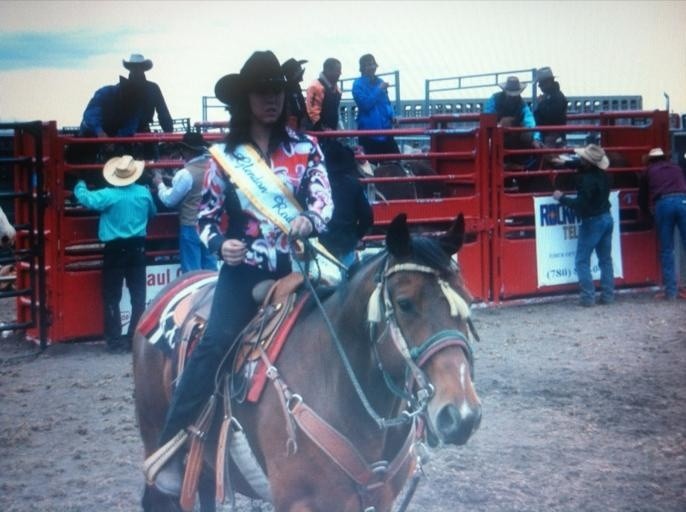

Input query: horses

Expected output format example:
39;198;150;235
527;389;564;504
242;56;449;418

131;212;483;512
359;162;451;236
498;112;638;238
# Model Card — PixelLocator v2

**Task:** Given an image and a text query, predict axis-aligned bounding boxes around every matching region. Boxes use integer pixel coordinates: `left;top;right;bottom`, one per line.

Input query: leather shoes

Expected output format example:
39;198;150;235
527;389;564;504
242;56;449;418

151;450;184;499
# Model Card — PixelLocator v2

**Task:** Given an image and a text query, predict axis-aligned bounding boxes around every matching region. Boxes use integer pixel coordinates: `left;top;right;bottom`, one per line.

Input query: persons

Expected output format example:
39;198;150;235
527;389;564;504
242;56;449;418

351;54;403;164
151;132;222;273
640;148;686;301
73;153;158;351
305;57;342;142
148;51;336;494
523;67;567;172
72;75;143;160
551;145;615;305
0;206;17;247
279;57;316;131
484;75;546;172
121;52;174;133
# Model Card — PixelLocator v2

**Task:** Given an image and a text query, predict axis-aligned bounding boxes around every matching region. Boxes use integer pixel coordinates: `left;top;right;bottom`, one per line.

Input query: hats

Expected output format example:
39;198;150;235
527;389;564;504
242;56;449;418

573;142;610;171
639;147;673;166
282;56;308;70
102;154;146;186
495;75;528;97
322;140;356;174
119;76;144;108
531;67;558;88
175;131;211;153
123;53;153;71
215;51;304;105
